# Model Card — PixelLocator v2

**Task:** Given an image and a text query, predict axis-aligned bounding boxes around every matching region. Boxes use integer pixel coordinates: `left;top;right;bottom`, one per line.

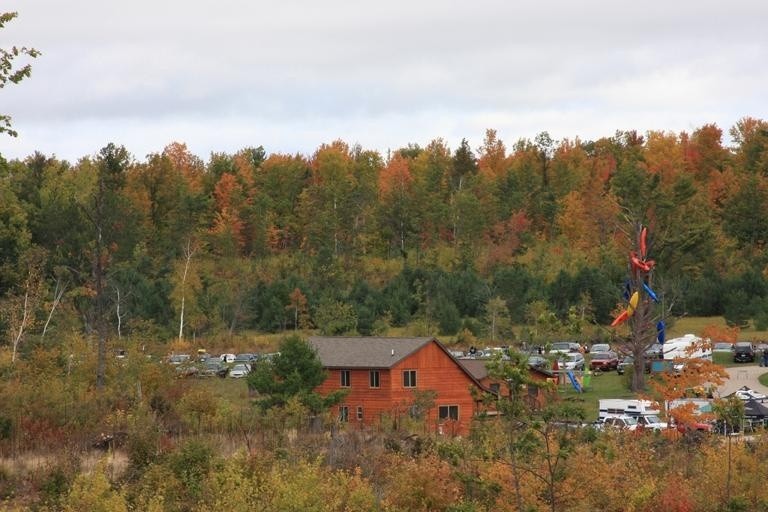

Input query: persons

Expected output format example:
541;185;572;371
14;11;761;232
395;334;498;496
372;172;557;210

469;346;476;355
711;387;720;399
652;352;656;360
658;348;664;359
580;344;585;353
583;342;590;353
538;345;542;354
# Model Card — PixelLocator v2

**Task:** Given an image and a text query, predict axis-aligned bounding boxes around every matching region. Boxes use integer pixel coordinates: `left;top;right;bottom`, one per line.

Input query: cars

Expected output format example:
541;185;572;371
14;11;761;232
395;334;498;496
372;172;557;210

530;396;718;437
